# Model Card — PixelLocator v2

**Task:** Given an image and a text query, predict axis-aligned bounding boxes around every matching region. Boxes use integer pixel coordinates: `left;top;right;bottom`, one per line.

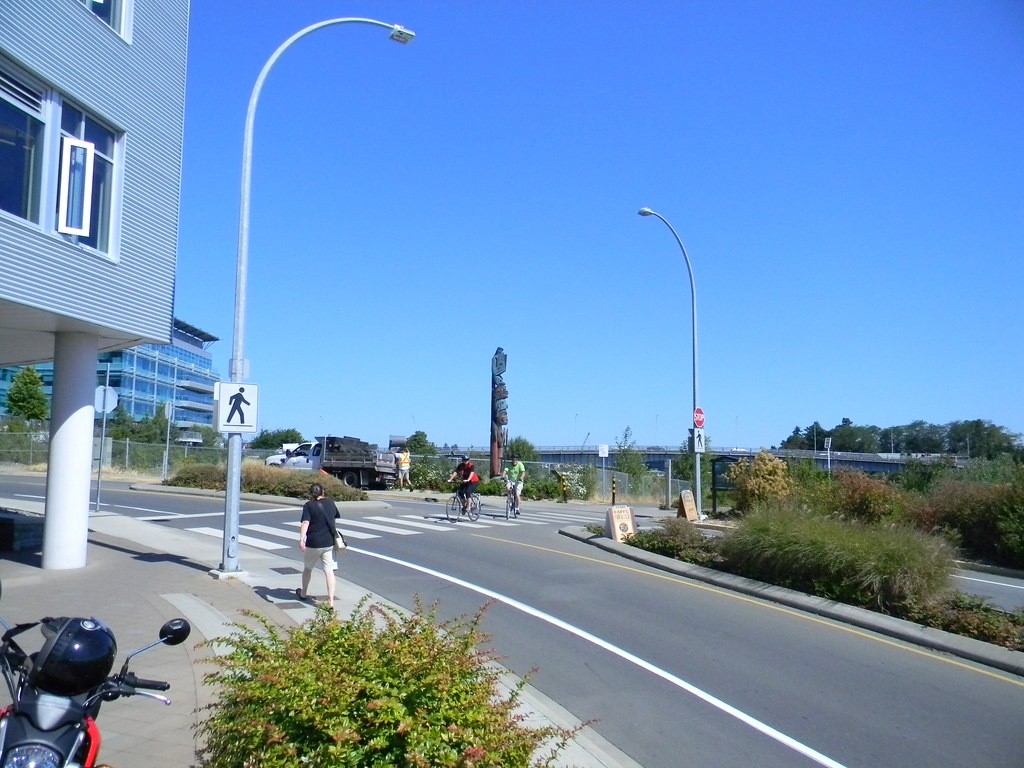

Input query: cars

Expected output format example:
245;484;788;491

265;454;287;466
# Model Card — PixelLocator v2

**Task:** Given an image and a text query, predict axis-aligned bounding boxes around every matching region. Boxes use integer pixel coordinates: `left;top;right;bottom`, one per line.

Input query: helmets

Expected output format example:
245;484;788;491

22;617;117;697
510;454;518;459
462;455;469;460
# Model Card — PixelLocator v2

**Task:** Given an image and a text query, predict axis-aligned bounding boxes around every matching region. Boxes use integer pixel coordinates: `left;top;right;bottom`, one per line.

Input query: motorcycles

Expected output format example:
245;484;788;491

0;580;191;768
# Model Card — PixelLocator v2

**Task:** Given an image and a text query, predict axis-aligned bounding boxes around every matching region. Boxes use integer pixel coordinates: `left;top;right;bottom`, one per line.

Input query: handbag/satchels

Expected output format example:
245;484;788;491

334;531;347;550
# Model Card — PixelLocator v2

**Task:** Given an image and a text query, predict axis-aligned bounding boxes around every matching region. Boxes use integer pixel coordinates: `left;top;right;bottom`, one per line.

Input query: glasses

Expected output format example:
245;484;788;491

512;458;517;460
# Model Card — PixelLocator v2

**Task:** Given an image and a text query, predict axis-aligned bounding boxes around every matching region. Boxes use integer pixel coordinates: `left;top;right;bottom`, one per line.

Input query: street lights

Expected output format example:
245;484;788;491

638;207;703;514
218;18;416;574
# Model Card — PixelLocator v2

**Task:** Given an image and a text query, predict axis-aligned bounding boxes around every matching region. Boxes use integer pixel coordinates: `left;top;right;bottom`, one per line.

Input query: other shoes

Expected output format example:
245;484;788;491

296;588;307;602
410;484;415;492
516;510;520;514
400;488;402;490
326;607;333;614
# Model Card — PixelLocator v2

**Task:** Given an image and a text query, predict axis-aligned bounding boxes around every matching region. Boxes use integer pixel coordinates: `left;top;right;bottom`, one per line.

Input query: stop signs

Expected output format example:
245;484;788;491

694;408;705;427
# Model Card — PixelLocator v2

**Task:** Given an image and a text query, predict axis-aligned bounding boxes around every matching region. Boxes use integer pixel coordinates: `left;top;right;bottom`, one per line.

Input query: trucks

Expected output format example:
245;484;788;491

280;436;409;490
275;443;299;453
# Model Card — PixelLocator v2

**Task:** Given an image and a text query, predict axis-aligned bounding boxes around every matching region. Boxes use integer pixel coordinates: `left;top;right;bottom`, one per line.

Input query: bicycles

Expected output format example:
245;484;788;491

446;481;481;523
504;479;519;520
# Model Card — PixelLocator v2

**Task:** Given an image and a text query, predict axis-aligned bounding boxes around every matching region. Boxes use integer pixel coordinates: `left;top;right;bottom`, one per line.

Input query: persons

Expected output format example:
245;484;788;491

447;455;480;511
295;484;341;614
501;454;526;515
390;447;415;492
